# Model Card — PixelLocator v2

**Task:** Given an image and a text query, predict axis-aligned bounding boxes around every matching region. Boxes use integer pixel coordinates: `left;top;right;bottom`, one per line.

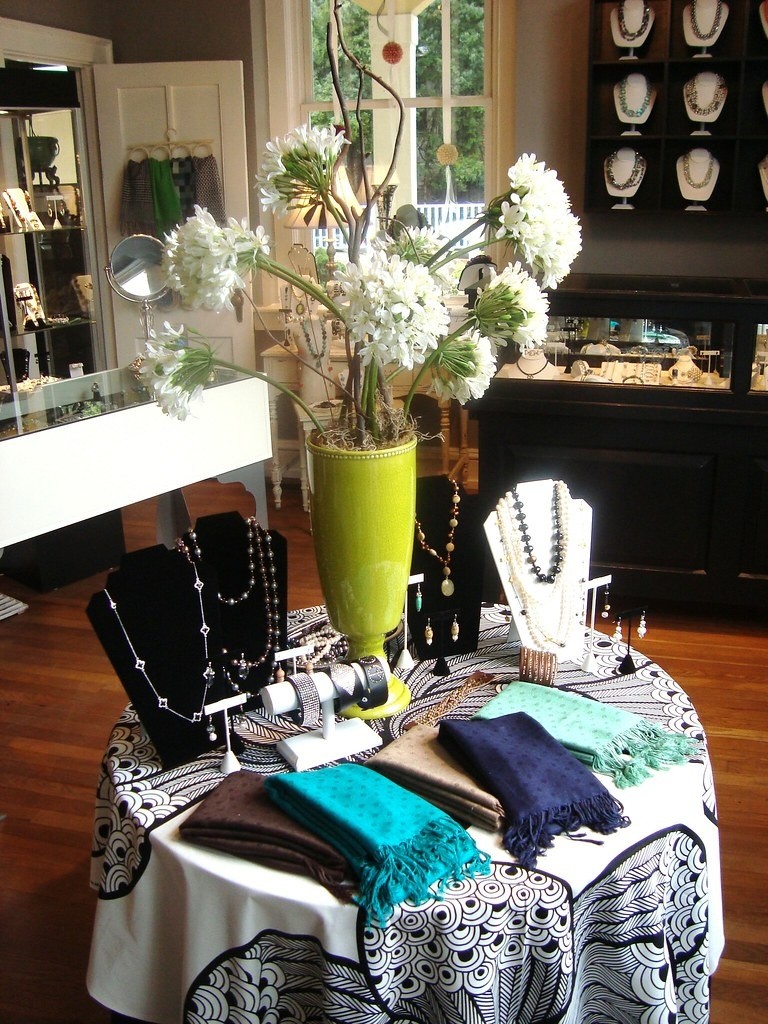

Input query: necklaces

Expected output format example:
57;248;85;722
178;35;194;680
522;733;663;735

619;78;654;118
606;151;645;190
682;152;715;189
290;284;307;314
675;363;694;377
617;2;650;42
15;284;43;321
100;535;216;724
495;479;587;663
2;187;33;222
685;73;727;116
690;0;723;40
184;515;286;713
3;352;29;383
515;358;550;379
74;275;90;305
415;474;462;597
300;317;328;369
282;619;350;673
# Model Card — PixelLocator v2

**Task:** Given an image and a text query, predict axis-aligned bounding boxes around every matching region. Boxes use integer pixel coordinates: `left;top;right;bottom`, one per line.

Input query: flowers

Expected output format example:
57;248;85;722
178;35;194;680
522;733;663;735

138;0;585;439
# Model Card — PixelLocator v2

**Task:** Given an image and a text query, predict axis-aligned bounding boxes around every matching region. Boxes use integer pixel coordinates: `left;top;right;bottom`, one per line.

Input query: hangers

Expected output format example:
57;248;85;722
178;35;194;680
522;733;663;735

128;127;214;161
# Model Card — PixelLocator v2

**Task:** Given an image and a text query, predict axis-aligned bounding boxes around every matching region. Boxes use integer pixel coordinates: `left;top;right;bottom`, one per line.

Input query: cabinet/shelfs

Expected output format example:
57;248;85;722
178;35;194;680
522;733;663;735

0;55;113;440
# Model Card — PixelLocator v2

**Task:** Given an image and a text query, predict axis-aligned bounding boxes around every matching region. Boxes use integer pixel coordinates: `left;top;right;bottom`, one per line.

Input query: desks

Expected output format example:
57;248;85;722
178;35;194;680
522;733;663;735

0;366;274;552
105;602;709;1024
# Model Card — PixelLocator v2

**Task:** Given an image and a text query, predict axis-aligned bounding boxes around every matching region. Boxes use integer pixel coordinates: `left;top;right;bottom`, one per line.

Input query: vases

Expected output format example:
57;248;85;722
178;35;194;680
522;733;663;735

305;428;418;720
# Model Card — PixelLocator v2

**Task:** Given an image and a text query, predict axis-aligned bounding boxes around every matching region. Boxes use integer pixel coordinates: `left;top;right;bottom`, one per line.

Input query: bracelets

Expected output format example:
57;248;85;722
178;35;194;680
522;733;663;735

285;671;319;727
322;662;365;713
517;647;558;687
352;654;389;710
